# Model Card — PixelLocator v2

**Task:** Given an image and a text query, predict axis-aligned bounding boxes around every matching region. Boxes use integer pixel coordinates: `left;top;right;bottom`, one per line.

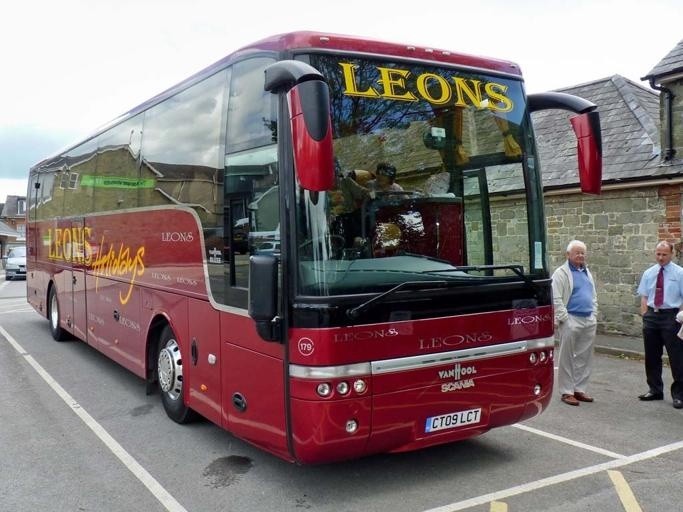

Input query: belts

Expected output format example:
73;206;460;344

648;307;677;313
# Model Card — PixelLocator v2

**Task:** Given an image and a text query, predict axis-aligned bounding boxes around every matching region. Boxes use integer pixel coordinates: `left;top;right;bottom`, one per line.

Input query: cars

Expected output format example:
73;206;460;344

5;248;27;279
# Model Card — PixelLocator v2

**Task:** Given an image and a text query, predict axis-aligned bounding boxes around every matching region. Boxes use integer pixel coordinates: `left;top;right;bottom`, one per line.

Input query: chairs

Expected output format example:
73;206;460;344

349;168;376;209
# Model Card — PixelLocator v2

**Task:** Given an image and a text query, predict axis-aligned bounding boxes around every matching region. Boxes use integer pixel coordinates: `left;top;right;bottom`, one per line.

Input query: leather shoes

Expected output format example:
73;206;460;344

673;398;682;409
637;391;664;400
561;392;594;406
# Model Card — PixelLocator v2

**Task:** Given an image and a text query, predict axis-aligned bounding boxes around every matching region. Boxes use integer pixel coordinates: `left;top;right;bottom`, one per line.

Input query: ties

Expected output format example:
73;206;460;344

654;267;665;307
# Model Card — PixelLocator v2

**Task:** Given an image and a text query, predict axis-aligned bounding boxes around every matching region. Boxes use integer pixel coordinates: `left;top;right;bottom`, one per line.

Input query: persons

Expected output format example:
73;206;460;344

359;159;409;218
633;239;682;411
549;238;599;407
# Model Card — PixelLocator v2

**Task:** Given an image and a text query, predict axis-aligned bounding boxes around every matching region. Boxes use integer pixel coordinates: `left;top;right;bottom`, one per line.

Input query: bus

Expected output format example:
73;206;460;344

24;34;604;470
